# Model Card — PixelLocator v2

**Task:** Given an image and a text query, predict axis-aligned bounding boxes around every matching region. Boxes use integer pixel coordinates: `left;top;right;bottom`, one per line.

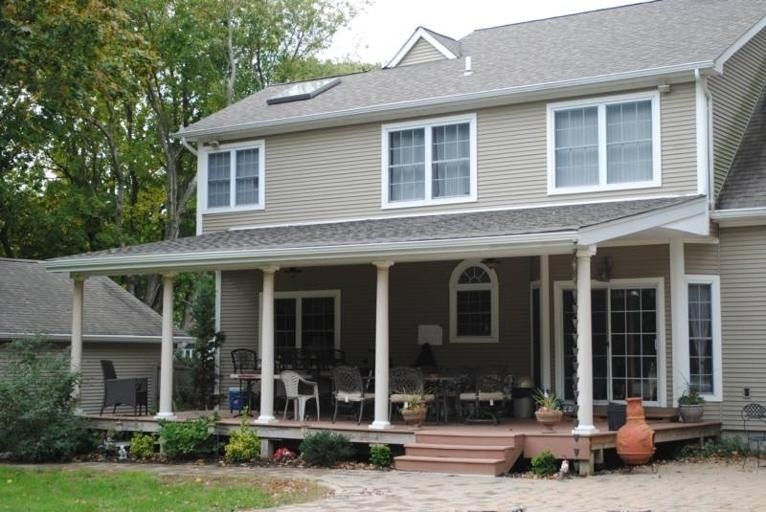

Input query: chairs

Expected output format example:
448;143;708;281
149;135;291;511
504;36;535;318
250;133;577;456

96;358;149;417
741;402;765;468
229;344;508;428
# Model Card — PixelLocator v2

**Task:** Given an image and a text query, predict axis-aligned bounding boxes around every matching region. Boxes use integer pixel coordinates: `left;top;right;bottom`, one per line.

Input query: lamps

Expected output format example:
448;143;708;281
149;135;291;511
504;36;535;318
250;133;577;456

480;260;502;270
284;268;303;278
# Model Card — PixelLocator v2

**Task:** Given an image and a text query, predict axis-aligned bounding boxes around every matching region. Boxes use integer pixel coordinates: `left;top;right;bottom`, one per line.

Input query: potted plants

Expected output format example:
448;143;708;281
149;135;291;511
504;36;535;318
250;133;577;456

531;389;564;434
677;390;704;422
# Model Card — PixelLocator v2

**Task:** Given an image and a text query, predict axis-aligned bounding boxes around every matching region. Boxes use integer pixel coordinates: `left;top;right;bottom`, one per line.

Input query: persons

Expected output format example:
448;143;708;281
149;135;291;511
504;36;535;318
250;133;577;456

413;343;435;374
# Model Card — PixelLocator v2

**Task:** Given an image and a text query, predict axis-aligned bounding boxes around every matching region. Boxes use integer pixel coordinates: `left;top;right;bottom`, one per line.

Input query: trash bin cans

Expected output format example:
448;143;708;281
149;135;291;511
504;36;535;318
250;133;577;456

513;376;534;418
229;388;246;415
608;401;627;430
